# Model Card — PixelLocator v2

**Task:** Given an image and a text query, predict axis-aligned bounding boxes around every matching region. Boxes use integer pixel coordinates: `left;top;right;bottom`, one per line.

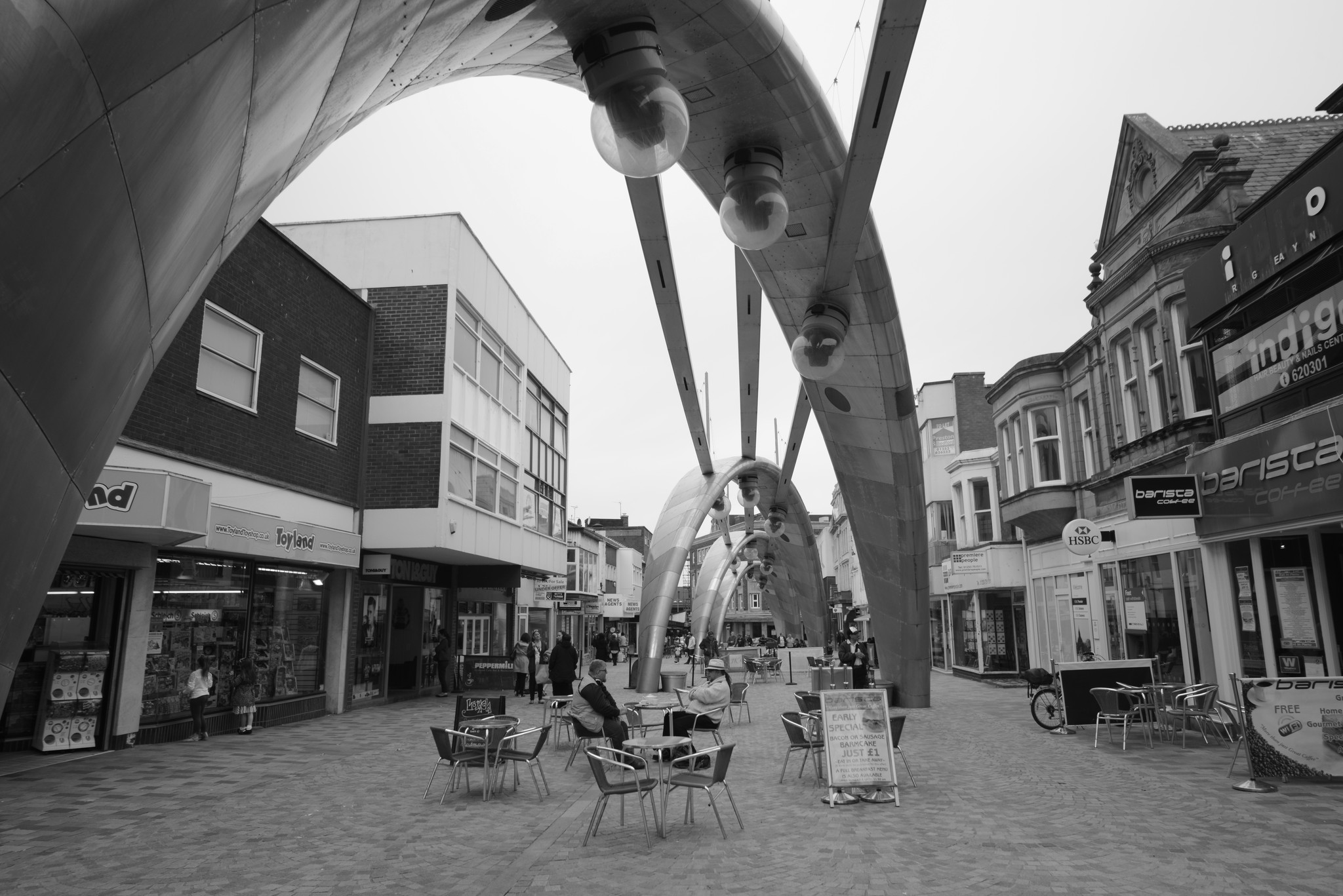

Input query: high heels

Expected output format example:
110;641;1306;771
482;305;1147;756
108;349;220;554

519;694;525;697
515;690;518;696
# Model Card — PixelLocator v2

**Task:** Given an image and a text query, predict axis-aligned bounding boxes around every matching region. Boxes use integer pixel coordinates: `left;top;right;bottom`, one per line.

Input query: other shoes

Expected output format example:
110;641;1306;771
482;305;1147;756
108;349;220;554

684;662;689;664
676;662;679;664
237;729;247;734
538;700;544;704
529;701;534;704
550;704;566;709
435;694;447;697
246;729;252;734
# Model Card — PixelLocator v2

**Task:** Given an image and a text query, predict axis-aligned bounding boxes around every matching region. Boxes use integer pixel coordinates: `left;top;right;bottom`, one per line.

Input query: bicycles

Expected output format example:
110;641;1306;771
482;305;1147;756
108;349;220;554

1030;651;1106;730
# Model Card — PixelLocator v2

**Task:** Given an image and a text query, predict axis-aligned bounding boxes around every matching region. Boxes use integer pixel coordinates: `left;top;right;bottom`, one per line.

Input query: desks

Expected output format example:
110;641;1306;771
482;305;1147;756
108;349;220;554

752;659;780;684
632;702;682;767
1142;683;1197;740
542;695;575;752
619;736;694;825
450;720;518;802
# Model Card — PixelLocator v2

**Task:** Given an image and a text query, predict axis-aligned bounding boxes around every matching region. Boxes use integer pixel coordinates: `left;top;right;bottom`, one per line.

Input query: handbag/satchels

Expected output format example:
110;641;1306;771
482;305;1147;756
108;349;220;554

535;661;552;684
618;652;623;662
605;640;611;656
672;746;710;768
510;644;517;661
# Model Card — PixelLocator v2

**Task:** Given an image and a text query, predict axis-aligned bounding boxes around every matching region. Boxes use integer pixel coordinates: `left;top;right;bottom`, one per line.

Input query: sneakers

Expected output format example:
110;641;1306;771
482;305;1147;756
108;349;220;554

624;758;648;770
652;748;670;761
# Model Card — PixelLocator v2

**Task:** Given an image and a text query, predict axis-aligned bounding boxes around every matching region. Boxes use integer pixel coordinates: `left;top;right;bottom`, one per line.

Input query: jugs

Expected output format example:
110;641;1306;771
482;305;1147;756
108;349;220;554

643;694;657;704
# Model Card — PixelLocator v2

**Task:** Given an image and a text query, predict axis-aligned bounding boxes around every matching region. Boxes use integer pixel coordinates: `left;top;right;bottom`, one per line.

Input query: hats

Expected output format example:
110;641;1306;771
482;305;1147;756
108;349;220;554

846;627;861;635
704;659;728;670
840;630;843;632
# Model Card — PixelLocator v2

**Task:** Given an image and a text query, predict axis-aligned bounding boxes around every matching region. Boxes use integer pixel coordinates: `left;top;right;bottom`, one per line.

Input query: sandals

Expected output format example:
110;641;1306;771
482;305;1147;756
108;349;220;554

199;735;208;740
182;736;199;742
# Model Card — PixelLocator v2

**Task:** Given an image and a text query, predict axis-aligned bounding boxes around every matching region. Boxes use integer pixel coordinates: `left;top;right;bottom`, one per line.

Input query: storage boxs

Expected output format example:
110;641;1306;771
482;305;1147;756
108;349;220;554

140;605;298;717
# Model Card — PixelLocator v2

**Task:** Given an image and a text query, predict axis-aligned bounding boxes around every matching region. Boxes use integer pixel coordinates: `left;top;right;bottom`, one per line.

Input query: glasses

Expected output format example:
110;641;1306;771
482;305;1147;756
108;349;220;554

707;669;717;672
532;629;538;635
596;669;606;674
239;658;245;663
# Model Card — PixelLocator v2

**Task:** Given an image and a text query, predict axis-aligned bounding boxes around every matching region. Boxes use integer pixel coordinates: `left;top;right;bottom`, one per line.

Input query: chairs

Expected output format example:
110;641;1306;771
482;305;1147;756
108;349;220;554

422;654;917;847
1089;681;1250;778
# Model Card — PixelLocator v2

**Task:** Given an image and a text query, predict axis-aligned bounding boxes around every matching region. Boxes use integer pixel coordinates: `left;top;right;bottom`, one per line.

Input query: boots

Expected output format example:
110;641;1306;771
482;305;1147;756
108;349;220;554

613;657;617;666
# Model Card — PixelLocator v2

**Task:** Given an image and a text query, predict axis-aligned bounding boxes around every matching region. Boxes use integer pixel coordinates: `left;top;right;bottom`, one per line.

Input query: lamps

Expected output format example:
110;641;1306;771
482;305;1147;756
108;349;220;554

313;572;329;585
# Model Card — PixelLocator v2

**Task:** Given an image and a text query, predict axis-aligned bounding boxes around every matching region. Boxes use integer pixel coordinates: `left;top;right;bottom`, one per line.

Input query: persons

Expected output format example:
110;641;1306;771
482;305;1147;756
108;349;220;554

285;645;293;657
229;658;256;734
652;659;732;763
361;596;381;650
699;630;719;667
168;658;178;673
548;630;579;709
828;640;832;646
777;633;807;648
867;636;875;644
727;631;753;647
838;627;869;689
513;632;531;696
284;662;292;674
664;632;695;664
592;629;626;666
179;655;213;742
526;629;552;704
836;630;845;647
759;634;767;646
570;659;648;770
434;628;452;698
286;679;294;691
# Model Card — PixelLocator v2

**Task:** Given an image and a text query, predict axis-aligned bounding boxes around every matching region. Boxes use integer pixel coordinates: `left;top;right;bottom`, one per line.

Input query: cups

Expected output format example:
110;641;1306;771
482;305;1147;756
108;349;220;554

652;699;658;705
641;700;648;704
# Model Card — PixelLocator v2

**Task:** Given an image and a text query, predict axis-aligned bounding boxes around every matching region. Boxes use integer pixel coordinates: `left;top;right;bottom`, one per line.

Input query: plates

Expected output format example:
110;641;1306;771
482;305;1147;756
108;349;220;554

639;703;648;706
